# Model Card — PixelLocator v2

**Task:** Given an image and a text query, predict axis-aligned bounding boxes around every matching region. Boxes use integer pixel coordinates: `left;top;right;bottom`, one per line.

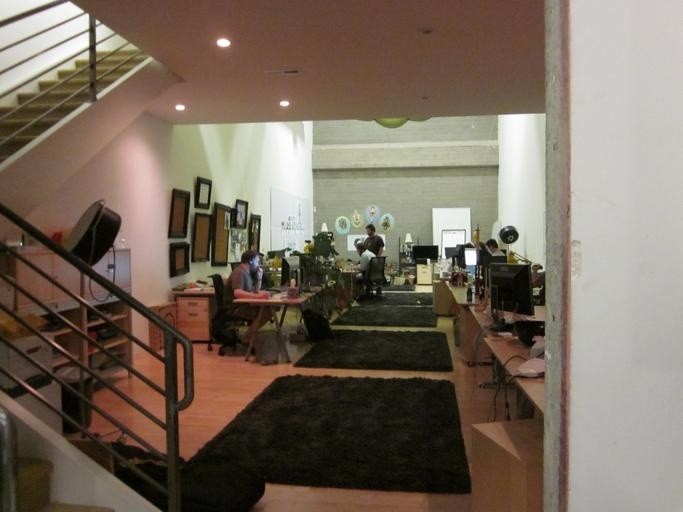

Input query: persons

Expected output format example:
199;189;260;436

221;249;275;350
484;238;506;257
362;224;384;297
348;242;380;300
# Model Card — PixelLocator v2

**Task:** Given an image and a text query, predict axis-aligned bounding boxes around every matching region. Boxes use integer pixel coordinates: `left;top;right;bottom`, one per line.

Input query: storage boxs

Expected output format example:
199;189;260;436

0;331;55;388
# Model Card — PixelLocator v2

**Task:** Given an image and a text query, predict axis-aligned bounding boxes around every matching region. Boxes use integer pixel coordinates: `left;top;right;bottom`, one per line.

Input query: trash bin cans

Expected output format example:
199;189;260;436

254;330;279;366
55;366;93;435
453;318;459;346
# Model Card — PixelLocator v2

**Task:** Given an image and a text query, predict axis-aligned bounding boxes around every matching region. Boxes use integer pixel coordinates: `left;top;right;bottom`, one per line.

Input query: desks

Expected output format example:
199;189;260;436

230;279;337;364
439;270;544;421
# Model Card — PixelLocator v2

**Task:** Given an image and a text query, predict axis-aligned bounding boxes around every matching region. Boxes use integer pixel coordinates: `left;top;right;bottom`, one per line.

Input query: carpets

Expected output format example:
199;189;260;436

292;327;452;374
353;292;432;307
189;373;473;497
372;284;415;292
331;308;437;327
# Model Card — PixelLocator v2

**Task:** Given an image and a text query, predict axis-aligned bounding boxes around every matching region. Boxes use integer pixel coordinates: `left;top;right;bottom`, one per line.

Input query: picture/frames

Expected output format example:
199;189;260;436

165;176;261;279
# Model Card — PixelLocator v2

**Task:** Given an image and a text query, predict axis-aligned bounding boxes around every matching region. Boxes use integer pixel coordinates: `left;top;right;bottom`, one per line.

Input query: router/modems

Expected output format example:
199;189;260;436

516;357;545;378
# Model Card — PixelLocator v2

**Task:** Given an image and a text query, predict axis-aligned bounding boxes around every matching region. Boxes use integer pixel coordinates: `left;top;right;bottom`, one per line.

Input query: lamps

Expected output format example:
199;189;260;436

499;225;518;263
61;197;123;446
404;232;414;257
320;222;328;233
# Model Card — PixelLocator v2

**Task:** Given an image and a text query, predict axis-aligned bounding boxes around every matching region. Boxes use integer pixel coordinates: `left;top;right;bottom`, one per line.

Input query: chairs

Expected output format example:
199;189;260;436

208;273;243;355
361;255;388;300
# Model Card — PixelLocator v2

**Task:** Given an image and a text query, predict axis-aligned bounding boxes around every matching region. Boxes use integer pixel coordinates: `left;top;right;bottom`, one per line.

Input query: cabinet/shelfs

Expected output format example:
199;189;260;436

468;419;544;512
417;264;433;285
433;280;459;315
176;295;212;342
37;308;84;374
86;248;133;306
0;248;82;315
86;298;134;384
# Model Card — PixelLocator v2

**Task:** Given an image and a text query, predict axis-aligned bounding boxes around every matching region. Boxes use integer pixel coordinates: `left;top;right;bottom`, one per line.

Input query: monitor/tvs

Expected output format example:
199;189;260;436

412;245;438;264
280;256;302;296
444;241;507;271
490;263;535;327
